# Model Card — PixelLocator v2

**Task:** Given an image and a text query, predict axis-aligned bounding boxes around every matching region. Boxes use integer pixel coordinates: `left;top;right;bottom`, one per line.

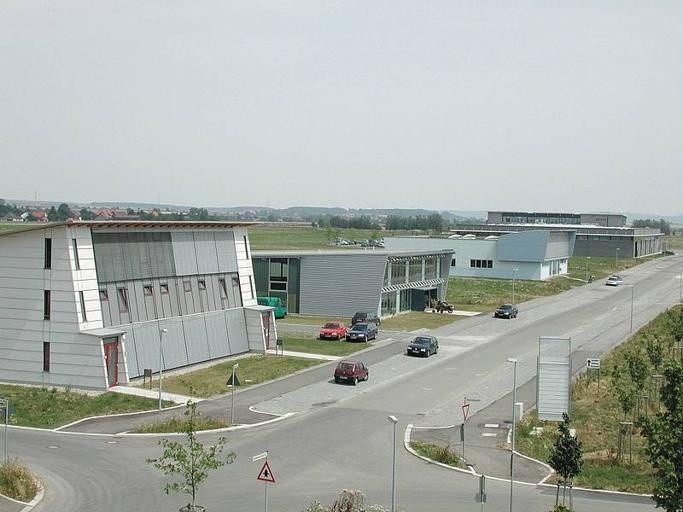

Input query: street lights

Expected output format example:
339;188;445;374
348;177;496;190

512;268;518;303
507;358;517;511
158;328;168;408
616;248;619;267
386;415;397;511
586;257;590;280
631;285;635;333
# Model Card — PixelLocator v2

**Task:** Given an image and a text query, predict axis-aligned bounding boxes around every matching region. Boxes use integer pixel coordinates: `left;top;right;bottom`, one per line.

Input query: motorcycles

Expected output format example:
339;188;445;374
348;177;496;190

436;301;455;313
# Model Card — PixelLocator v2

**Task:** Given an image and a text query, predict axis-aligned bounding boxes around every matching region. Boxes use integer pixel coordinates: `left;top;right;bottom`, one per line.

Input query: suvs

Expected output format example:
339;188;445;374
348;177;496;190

257;296;287;319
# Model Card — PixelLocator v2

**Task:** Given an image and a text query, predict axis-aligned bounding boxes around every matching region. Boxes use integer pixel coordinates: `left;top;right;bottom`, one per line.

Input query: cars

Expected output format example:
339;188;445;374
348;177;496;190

352;311;381;326
319;320;346;340
407;336;439;357
605;277;623;285
494;304;518;319
334;358;369;385
346;323;378;342
334;234;500;248
